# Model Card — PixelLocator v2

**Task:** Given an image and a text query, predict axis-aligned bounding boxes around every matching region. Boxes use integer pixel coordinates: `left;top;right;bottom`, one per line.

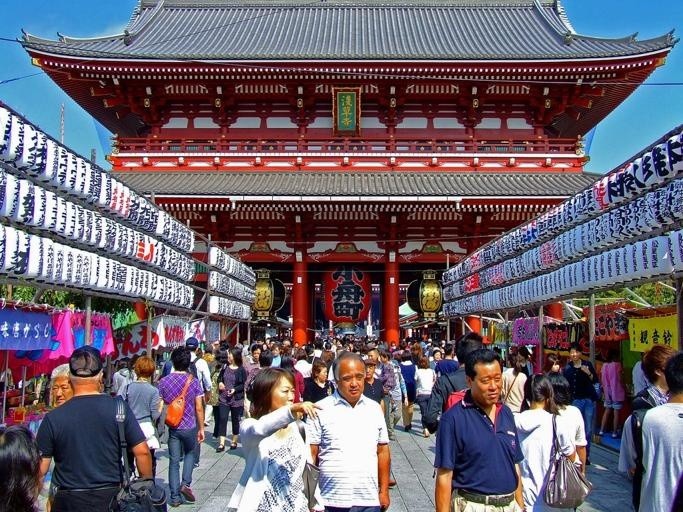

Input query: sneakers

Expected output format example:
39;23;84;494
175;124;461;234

378;479;396;489
611;434;618;439
595;431;603;436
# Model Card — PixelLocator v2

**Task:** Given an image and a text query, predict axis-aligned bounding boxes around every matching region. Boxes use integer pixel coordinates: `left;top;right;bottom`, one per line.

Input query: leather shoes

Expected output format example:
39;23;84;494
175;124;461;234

179;484;196;502
172;498;182;507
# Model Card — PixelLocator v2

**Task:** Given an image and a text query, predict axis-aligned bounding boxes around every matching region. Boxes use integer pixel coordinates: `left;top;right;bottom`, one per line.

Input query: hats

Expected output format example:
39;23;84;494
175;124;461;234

186;336;199;348
364;358;377;366
69;345;103;377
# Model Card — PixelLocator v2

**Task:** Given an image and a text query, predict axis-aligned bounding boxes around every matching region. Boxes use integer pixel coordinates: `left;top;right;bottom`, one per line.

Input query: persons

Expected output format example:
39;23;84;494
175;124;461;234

200;330;458;356
617;350;683;512
509;373;577;512
367;347;396;437
157;347;205;507
434;349;526;511
501;353;528;413
365;358;386;418
389;361;408;427
546;374;588;478
562;342;598;465
1;423;44;512
226;366;323;512
244;350;273;419
424;332;483;436
414;355;437;438
517;347;533;410
303;359;335;405
186;336;213;468
280;354;305;404
544;353;562;374
376;349;397;390
32;346;152;512
434;344;460;377
399;350;418;432
215;347;247;453
121;356;164;479
306;352;392;512
429;350;439;368
48;362;73;408
637;343;678;408
599;349;627;439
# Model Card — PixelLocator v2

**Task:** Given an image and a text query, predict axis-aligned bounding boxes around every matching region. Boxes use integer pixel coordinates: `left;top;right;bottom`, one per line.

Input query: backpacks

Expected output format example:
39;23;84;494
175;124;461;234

188;356;202;380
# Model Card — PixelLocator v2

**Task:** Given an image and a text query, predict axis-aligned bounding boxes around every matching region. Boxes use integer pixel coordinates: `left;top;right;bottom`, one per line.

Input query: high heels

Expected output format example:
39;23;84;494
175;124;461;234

230;442;238;449
216;443;226;451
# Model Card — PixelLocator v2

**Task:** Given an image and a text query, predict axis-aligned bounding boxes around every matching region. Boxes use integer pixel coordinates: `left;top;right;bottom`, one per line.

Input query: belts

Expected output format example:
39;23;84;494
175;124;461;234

457;488;514;507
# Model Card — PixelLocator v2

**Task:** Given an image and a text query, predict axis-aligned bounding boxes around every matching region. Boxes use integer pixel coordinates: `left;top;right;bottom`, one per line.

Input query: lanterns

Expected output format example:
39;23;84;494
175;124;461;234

0;102;259;321
248;268;287;324
322;264;372;329
406;269;444;323
440;128;683;321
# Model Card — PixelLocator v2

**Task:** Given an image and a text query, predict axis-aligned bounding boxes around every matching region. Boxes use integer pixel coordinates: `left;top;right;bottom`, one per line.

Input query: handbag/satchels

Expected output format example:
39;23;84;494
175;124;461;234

108;395;168;512
585;359;603;400
164;373;194;428
542;412;594;509
296;419;322;509
